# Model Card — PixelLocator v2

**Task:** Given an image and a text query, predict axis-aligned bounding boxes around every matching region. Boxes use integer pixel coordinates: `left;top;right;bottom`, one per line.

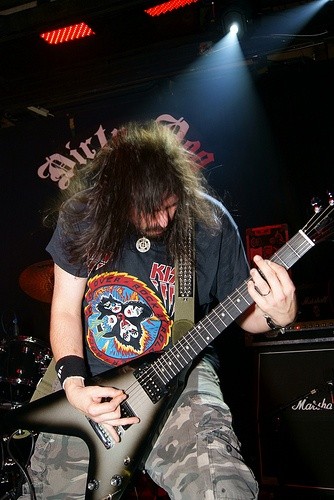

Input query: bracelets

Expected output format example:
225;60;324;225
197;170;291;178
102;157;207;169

264;314;298;335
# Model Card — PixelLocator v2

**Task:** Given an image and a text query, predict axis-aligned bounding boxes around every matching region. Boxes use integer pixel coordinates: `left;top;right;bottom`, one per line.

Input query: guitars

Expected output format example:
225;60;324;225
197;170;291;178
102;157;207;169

0;192;334;500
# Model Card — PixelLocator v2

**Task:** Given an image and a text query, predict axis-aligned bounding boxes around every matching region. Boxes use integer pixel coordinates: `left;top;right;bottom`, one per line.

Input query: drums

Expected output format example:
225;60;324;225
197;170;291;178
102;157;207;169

0;393;28;440
1;334;53;390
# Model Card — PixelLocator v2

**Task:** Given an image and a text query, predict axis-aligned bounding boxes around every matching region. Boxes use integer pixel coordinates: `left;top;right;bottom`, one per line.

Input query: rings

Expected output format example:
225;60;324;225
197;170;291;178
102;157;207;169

260;290;271;296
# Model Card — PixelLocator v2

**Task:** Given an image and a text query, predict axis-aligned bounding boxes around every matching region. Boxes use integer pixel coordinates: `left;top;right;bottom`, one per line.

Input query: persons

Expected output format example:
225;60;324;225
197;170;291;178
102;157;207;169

18;123;296;500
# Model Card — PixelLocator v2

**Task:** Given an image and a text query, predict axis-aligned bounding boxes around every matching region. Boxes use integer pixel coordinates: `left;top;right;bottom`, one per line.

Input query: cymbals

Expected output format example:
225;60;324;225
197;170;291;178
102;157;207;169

16;256;56;309
